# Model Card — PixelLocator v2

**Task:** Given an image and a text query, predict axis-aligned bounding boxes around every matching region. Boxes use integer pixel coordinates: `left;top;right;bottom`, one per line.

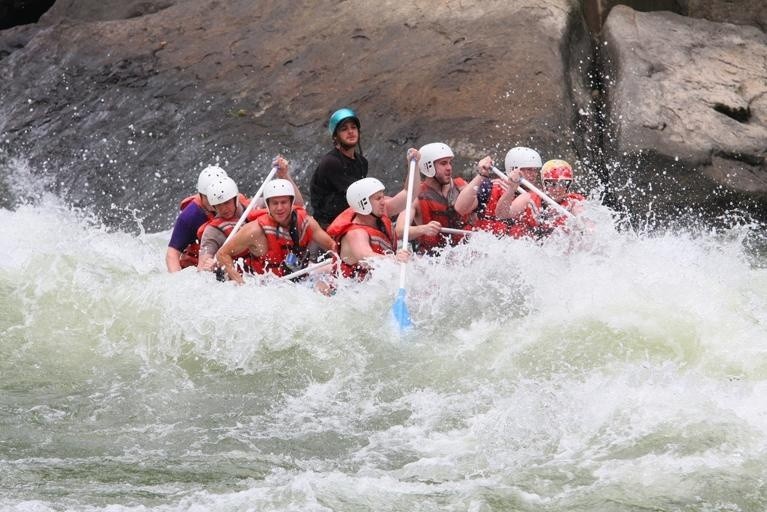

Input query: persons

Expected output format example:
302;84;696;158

495;159;587;242
326;147;421;282
310;107;368;231
453;148;541;238
197;155;304;271
215;179;339;297
166;165;246;274
394;142;468;256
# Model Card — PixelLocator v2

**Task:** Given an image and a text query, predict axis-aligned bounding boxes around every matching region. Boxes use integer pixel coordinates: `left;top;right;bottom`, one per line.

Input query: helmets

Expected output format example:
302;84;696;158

505;147;542;177
206;176;239;207
346;177;385;215
198;166;228;195
329;108;360;142
541;159;573;193
418;142;455;178
263;179;295;214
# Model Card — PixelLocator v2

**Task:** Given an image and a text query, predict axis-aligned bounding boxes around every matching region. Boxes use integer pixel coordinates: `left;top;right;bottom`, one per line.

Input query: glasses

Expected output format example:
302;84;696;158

544;180;571;193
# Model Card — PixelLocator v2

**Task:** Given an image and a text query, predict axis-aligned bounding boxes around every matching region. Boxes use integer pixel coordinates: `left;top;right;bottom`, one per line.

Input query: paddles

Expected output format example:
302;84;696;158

392;158;416;333
